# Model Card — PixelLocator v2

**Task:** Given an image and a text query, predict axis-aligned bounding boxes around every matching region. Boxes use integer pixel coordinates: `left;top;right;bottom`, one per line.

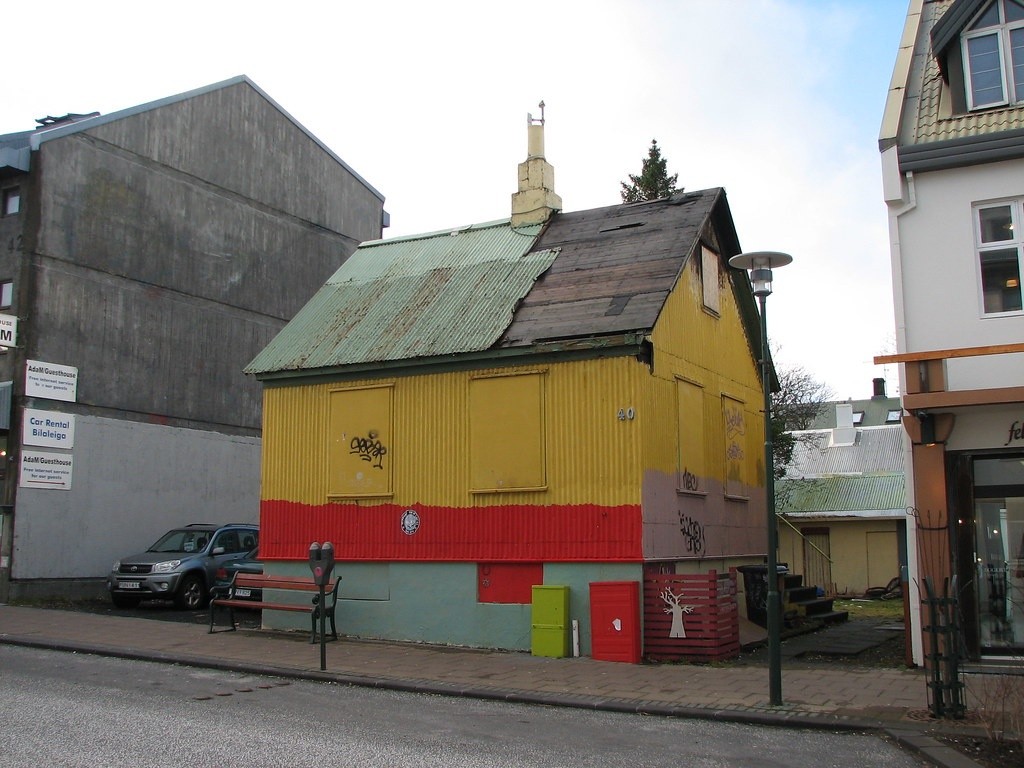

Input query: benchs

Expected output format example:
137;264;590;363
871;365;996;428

206;570;343;644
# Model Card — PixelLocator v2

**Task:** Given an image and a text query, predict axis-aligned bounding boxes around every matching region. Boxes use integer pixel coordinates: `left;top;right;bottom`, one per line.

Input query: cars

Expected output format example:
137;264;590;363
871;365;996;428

215;546;262;603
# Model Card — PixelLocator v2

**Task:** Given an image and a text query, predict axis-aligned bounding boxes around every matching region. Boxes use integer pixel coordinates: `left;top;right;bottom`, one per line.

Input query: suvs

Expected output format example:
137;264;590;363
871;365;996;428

105;523;260;610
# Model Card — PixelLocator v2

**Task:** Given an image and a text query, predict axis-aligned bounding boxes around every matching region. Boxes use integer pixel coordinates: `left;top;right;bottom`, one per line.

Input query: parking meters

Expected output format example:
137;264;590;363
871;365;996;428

307;541;334;670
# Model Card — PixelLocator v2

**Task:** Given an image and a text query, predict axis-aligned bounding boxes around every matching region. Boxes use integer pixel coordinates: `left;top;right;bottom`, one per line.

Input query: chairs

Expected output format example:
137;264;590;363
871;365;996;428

195;536;254;555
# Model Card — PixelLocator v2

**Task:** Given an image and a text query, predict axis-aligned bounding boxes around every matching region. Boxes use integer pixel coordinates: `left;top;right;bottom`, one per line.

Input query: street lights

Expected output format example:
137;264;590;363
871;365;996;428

724;247;793;705
